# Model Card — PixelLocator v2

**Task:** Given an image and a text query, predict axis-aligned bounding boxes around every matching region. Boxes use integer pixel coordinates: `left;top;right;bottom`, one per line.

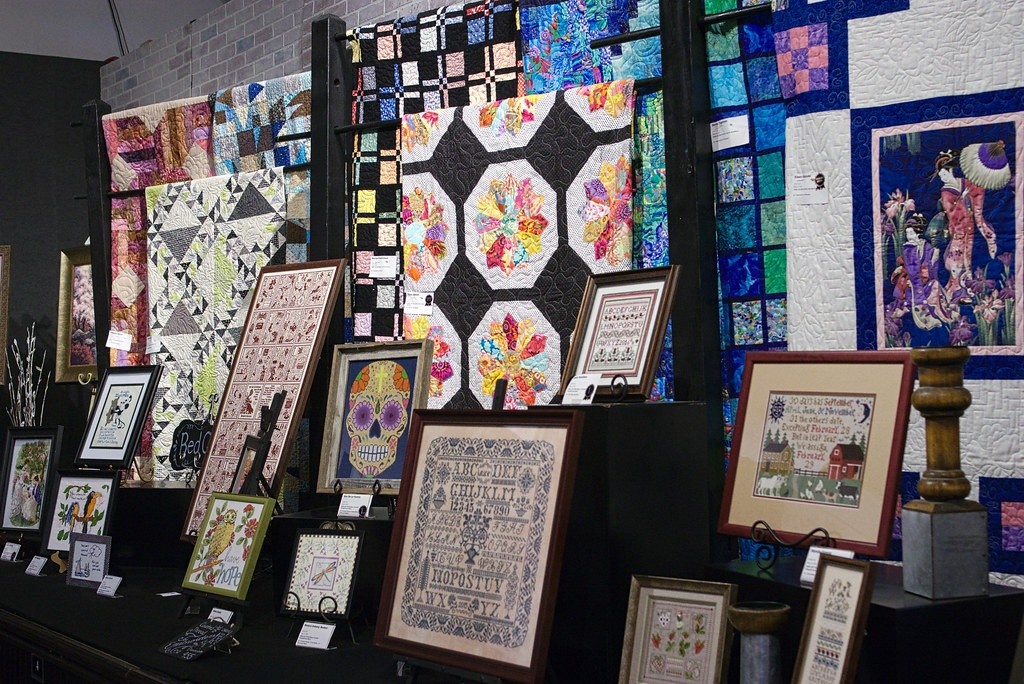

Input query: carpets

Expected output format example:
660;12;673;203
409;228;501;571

102;71;312;514
344;0;1024;590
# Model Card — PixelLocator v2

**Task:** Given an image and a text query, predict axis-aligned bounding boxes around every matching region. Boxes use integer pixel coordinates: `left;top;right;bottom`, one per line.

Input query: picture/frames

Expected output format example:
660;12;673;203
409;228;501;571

0;244;12;385
40;469;122;558
717;350;916;559
67;532;112;589
0;425;66;537
180;259;348;541
281;529;365;618
557;265;681;398
55;245;98;383
792;553;875;684
618;574;740;684
73;364;164;468
373;408;585;684
316;339;434;496
182;492;276;600
